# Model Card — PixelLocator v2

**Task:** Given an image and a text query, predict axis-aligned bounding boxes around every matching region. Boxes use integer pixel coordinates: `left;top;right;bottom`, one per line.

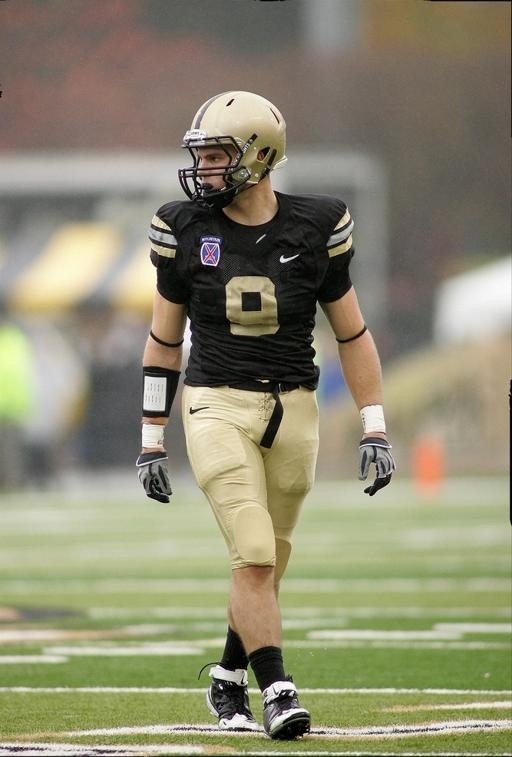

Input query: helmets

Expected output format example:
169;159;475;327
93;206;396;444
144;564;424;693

186;89;288;185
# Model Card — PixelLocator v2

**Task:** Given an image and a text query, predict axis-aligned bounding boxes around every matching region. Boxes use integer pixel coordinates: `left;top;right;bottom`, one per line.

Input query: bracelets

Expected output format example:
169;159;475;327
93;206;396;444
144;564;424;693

140;365;180;417
359;404;387;434
141;423;166;451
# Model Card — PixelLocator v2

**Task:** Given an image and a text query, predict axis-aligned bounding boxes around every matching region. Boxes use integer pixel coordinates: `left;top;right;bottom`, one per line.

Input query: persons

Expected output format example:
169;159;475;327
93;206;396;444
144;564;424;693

24;298;142;492
136;91;397;742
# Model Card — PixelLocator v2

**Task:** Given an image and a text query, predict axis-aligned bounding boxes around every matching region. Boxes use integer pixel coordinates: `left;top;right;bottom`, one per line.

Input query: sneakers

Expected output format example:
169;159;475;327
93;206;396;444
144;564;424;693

263;681;311;738
206;663;263;733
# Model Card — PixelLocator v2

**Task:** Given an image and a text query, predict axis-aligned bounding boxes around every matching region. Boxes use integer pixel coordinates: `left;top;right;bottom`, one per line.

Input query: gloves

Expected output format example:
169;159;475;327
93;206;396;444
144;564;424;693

357;437;397;497
136;451;173;504
